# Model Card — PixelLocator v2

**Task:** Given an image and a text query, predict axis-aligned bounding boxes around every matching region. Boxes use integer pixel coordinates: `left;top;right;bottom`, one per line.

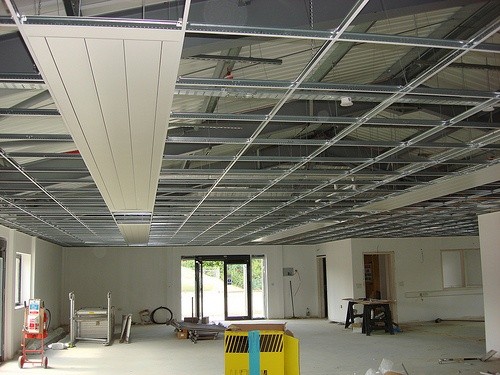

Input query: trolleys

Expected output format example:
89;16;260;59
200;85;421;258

18;300;48;367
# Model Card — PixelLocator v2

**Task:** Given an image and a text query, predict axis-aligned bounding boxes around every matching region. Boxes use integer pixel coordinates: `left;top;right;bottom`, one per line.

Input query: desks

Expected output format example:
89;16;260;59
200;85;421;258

343;299;397;335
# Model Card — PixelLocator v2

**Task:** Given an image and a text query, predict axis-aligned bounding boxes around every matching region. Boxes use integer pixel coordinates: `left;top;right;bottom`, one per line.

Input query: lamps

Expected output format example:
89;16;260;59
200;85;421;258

340;97;352;107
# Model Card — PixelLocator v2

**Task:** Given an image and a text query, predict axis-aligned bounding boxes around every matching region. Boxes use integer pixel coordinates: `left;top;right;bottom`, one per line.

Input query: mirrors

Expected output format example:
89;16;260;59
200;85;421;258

440;248;481;289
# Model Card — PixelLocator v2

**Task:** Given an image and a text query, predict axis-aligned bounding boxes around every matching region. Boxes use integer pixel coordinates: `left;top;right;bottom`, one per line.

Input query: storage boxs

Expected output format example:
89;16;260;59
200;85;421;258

203;317;209;323
224;324;303;375
184;317;199;323
